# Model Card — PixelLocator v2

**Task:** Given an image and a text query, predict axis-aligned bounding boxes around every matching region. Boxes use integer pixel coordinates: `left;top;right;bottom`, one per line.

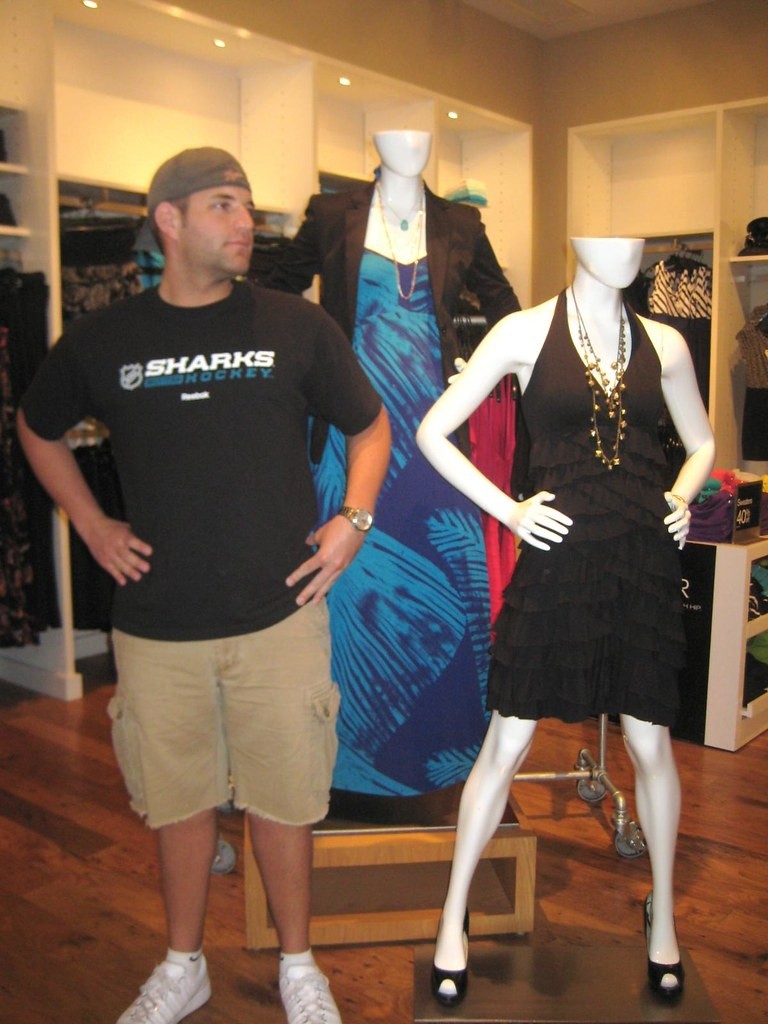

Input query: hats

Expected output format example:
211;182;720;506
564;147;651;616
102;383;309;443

738;217;768;256
131;147;251;251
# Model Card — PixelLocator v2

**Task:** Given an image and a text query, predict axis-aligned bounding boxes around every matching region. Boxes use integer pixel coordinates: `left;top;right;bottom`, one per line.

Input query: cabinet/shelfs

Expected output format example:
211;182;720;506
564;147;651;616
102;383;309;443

0;0;533;701
566;96;768;753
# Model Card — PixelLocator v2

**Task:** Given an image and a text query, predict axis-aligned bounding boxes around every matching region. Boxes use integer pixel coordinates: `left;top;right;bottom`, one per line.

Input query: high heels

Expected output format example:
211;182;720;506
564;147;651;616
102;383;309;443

643;890;684;1001
431;905;470;1006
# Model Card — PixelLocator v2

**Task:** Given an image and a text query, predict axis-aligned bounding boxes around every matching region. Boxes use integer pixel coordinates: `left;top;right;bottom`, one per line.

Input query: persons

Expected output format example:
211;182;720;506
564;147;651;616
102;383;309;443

19;146;391;1024
254;131;522;796
416;236;717;1007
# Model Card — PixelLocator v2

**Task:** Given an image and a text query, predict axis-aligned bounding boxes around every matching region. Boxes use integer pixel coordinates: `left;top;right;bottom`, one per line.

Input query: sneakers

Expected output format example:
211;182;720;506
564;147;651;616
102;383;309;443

116;954;211;1024
279;963;343;1024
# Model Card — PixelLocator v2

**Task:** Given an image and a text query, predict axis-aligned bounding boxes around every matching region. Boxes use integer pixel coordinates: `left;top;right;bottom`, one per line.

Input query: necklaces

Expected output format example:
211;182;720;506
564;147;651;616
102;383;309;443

571;280;628;470
376;175;424;300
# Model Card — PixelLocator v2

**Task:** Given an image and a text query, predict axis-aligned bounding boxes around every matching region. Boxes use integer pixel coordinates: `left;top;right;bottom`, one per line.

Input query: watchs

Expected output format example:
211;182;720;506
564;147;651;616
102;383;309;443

338;506;373;532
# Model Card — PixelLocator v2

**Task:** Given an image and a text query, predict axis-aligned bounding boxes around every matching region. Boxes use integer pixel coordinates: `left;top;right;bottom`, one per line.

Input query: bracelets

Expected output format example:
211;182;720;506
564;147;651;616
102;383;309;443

673;495;686;503
